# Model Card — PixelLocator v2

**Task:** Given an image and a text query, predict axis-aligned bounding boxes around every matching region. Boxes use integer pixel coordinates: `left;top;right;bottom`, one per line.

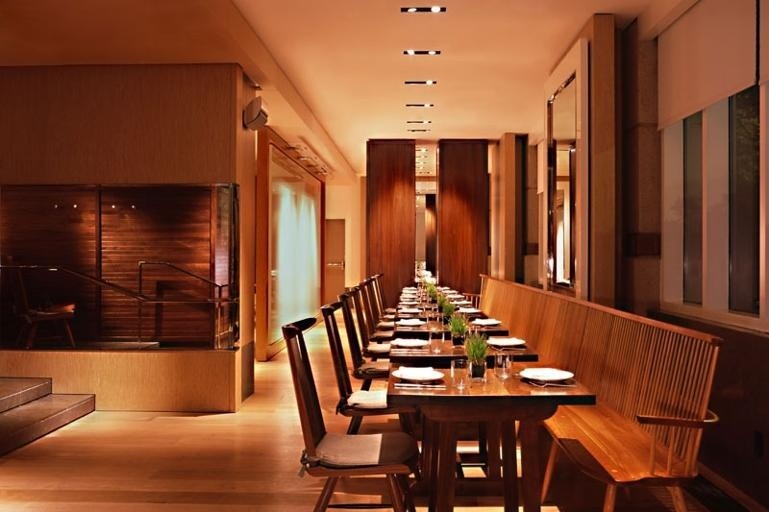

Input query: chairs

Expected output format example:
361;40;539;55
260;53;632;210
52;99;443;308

320;300;421;481
281;317;420;511
337;273;396;390
6;265;79;350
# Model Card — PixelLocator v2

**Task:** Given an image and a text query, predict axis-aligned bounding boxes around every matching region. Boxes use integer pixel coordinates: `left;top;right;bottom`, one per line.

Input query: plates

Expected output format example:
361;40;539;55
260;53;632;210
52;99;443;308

441;286;577;384
391;285;445;383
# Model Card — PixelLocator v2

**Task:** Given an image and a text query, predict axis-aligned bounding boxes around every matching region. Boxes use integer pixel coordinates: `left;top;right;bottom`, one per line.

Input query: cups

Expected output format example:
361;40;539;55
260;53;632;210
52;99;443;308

418;288;512;390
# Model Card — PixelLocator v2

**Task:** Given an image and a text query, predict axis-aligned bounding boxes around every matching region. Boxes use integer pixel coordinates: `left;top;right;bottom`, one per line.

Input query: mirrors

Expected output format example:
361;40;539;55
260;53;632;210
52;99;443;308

547;70;578;292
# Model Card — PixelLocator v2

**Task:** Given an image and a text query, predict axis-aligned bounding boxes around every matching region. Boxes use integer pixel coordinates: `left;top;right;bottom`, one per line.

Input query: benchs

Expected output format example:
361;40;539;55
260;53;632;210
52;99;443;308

462;272;726;512
